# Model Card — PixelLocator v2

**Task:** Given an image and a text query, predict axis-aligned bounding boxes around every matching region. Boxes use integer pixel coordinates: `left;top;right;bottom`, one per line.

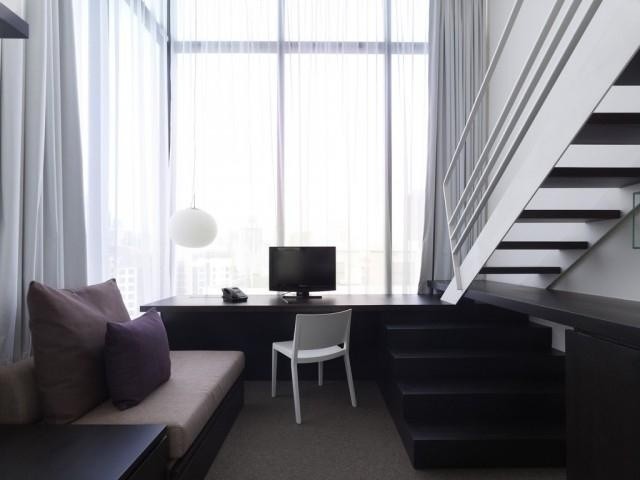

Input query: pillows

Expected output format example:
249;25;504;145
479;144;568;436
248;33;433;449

27;275;173;427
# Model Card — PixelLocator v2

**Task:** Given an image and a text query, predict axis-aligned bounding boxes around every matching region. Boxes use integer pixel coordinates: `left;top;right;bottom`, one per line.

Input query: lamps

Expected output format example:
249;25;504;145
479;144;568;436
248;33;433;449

165;0;219;250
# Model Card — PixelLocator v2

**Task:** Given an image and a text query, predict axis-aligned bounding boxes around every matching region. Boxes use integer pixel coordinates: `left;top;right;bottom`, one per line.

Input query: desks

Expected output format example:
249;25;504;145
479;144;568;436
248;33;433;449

139;291;461;381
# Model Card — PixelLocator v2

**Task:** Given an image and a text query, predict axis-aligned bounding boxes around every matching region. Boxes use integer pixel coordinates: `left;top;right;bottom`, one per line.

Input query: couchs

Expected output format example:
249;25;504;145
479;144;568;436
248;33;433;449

0;344;244;479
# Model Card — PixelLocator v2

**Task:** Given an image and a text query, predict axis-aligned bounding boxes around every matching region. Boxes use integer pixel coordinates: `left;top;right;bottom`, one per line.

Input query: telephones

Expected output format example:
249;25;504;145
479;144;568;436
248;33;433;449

222;286;248;303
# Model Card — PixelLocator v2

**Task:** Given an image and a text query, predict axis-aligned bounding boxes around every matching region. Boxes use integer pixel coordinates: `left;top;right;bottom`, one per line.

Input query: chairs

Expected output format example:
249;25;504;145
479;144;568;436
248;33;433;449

268;308;357;427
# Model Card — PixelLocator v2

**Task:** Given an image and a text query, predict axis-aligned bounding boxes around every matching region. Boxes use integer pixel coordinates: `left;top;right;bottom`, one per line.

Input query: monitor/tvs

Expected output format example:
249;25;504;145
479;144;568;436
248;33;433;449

268;246;336;299
